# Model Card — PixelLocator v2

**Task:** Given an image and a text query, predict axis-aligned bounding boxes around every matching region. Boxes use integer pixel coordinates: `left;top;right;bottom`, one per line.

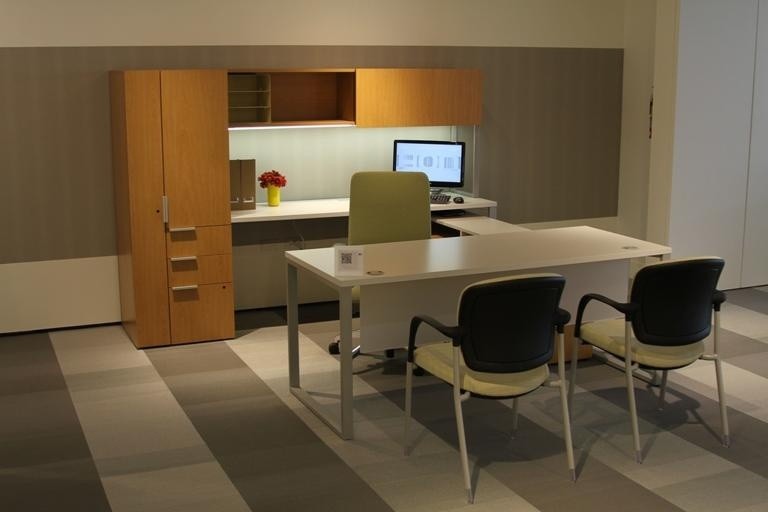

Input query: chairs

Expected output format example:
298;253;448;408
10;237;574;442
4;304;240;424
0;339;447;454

403;272;577;503
566;254;732;465
326;171;432;362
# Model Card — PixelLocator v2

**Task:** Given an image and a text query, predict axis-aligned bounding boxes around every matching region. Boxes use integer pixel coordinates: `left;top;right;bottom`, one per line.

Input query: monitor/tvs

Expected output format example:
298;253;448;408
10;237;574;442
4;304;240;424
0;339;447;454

392;139;466;190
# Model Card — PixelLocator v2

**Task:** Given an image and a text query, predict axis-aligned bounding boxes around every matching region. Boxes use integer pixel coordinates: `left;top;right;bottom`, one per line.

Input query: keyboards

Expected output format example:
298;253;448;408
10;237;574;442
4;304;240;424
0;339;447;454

430;193;450;204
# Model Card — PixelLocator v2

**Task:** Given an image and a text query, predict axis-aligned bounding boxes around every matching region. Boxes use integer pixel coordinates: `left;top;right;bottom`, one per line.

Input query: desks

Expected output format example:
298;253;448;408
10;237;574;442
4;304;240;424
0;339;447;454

431;209;533;236
283;225;671;442
231;191;498;224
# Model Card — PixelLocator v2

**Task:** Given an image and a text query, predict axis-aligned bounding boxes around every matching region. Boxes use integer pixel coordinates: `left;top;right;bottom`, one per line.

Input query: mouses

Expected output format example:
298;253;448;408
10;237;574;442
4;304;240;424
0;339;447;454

453;196;465;204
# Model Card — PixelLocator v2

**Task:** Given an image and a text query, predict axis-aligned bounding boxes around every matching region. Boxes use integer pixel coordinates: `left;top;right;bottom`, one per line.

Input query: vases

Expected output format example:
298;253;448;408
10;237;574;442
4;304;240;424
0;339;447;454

268;185;280;206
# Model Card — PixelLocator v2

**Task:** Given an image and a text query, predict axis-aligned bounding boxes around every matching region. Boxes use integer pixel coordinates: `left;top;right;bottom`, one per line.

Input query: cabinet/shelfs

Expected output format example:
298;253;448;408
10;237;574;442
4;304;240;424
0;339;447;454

112;69;237;348
227;68;482;127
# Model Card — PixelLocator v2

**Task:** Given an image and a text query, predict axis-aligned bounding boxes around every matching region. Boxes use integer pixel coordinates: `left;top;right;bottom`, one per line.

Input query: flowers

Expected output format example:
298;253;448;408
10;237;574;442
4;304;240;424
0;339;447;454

258;170;287;190
